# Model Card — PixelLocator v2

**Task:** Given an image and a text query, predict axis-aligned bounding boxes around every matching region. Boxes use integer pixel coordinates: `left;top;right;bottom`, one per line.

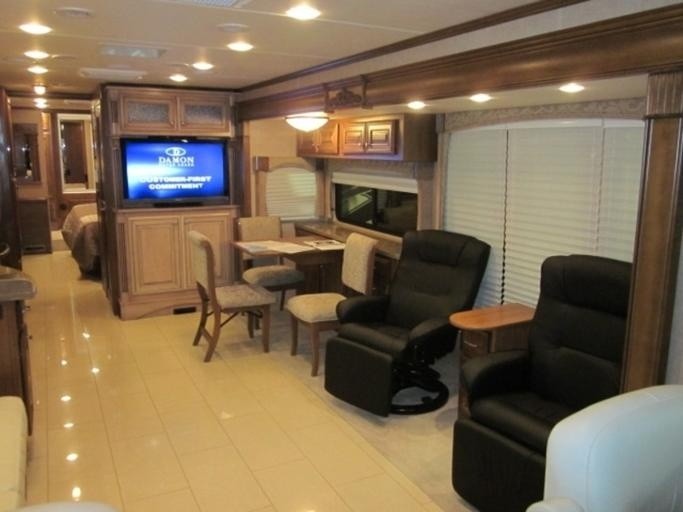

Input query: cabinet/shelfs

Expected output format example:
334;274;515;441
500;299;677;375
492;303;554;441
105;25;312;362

113;203;237;321
0;301;34;436
448;303;537;415
294;114;437;163
116;88;231;136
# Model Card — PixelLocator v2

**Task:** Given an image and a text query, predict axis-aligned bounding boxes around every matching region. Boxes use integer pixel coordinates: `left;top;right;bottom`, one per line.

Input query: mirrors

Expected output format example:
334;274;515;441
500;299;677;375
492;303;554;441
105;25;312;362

55;113;97;194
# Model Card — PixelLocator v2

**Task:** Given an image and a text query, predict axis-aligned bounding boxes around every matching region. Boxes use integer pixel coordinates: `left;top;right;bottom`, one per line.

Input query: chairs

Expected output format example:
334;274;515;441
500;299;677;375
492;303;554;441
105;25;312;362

188;214;378;377
322;228;492;417
451;252;683;511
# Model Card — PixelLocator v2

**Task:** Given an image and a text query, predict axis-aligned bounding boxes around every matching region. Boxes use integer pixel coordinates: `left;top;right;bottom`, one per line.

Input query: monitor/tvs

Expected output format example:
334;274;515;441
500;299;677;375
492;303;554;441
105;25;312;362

119;137;230;207
